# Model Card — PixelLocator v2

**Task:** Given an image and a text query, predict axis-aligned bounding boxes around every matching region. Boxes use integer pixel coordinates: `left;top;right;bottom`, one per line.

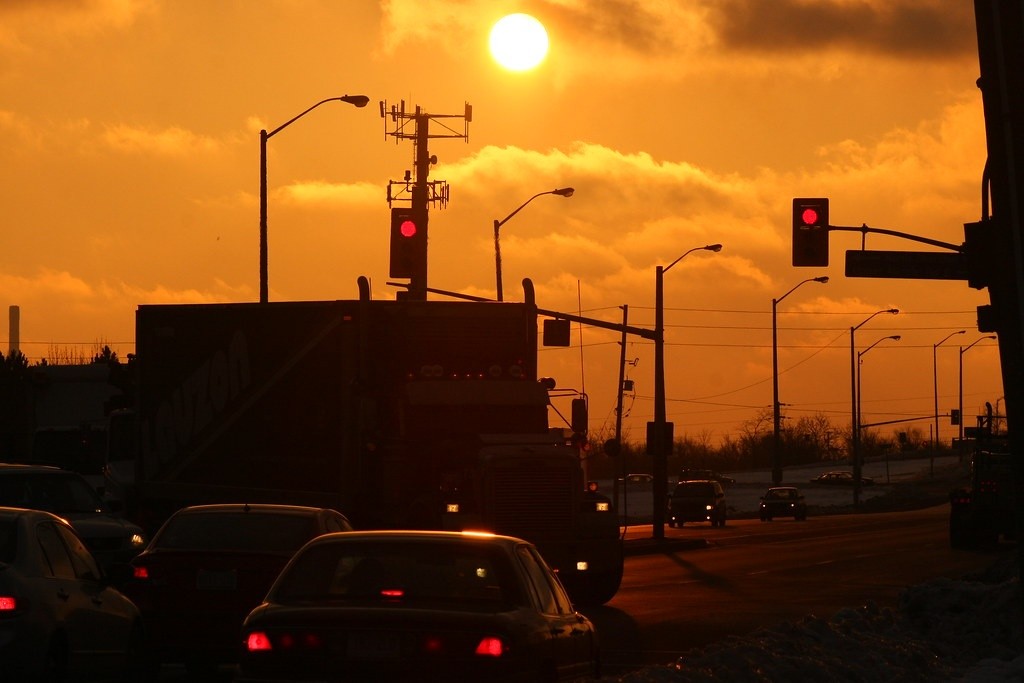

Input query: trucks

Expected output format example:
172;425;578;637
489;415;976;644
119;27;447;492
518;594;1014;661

134;300;625;605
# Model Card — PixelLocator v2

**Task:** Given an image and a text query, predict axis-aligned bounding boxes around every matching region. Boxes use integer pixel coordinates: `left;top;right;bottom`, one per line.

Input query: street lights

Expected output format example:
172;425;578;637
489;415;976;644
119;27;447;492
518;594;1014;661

493;187;577;303
857;334;902;446
256;93;370;303
958;335;997;440
650;243;726;539
771;275;829;465
934;330;966;450
849;307;901;450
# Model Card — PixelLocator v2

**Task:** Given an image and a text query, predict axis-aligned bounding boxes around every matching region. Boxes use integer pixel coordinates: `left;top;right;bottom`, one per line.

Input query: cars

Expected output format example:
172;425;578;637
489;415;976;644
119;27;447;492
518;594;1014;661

677;468;736;484
617;472;652;484
0;457;372;683
809;472;876;489
668;479;725;530
758;487;806;521
231;529;608;683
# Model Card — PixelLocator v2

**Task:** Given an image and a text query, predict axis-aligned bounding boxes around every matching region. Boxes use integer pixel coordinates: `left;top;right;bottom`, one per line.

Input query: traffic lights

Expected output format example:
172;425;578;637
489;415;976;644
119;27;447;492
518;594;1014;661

389;206;429;279
792;197;830;268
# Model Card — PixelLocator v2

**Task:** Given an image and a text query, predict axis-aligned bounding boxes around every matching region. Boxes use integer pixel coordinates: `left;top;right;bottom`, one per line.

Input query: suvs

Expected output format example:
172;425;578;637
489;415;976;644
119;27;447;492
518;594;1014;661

943;443;1009;553
